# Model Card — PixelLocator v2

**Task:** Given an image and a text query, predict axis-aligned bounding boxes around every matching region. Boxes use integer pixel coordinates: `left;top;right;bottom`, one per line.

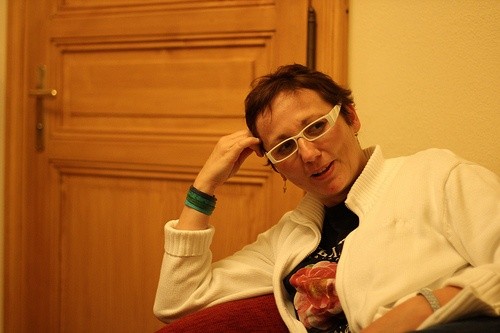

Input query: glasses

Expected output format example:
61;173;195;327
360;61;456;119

264;101;342;164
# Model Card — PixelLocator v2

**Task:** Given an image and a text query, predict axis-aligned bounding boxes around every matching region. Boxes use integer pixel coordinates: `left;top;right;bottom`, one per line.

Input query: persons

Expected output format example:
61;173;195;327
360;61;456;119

152;60;500;332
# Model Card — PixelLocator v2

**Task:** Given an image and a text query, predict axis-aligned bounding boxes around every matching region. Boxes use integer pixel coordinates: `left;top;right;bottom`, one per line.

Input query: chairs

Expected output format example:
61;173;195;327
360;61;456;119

155;293;291;333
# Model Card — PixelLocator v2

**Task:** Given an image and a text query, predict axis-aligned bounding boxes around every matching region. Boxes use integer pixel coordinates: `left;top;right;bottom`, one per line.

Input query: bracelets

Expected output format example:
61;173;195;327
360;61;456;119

185;184;217;216
416;288;442;312
